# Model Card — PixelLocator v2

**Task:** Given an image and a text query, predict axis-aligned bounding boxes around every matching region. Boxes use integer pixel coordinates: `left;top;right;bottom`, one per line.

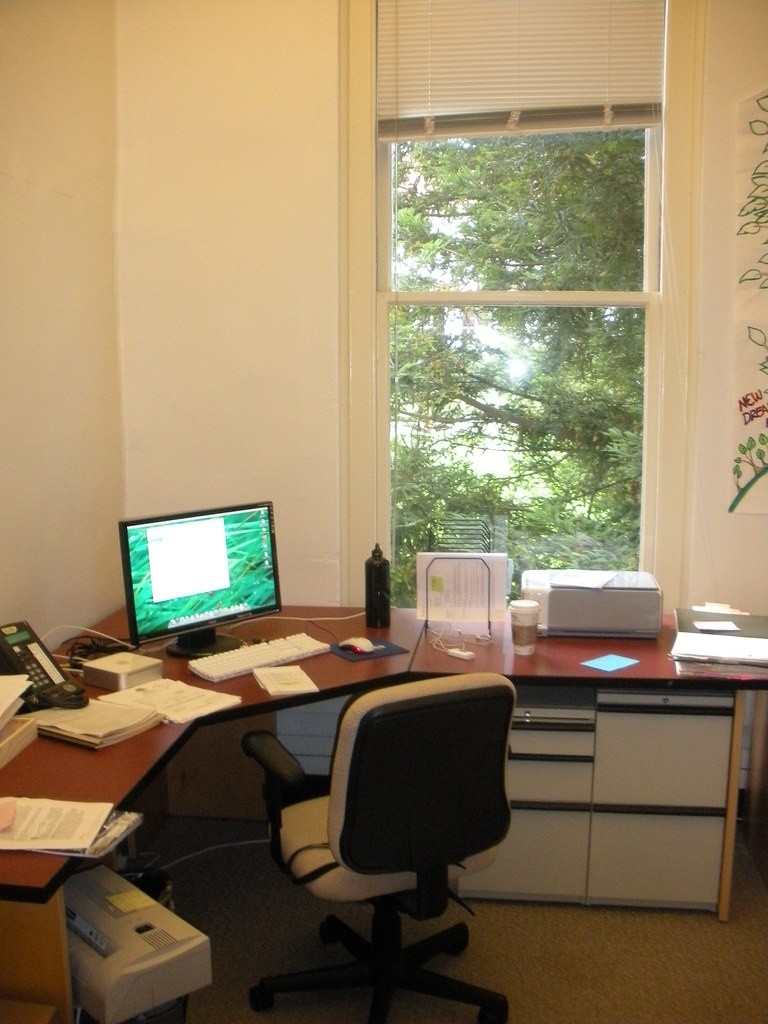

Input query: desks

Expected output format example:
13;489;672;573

0;607;768;1024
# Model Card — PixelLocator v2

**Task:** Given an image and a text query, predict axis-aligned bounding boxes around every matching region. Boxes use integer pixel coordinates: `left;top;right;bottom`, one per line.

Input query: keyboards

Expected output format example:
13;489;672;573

188;633;332;683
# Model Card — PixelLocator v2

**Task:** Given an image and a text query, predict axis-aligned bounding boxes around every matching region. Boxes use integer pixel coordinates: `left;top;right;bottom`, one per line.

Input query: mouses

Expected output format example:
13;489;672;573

339;636;375;654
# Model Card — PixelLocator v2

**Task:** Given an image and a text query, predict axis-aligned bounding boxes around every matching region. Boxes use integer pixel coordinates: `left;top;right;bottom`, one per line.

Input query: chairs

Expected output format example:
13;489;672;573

245;675;516;1024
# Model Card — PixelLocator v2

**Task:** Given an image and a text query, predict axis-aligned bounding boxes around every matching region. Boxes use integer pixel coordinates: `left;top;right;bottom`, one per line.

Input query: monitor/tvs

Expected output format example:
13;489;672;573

116;501;282;658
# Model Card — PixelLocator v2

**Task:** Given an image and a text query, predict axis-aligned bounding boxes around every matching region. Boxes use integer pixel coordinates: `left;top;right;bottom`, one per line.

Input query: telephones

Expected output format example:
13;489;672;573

0;619;87;714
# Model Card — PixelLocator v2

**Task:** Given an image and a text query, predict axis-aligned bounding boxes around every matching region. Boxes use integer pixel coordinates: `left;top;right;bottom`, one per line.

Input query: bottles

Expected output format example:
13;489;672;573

365;543;391;629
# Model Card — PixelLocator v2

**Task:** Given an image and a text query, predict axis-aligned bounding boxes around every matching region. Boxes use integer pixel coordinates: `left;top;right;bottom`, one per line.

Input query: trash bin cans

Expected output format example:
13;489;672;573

116;868;175;913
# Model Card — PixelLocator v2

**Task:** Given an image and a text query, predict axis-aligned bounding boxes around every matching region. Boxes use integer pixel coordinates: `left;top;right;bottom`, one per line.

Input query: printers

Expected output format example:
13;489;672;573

520;570;664;638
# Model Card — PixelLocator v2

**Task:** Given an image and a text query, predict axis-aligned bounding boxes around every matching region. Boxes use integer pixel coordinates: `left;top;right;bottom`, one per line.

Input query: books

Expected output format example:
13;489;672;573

668;607;768;678
25;809;144;858
15;698;166;749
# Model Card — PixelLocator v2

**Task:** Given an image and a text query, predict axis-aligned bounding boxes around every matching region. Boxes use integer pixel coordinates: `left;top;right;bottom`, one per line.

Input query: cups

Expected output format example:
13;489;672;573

508;600;541;655
